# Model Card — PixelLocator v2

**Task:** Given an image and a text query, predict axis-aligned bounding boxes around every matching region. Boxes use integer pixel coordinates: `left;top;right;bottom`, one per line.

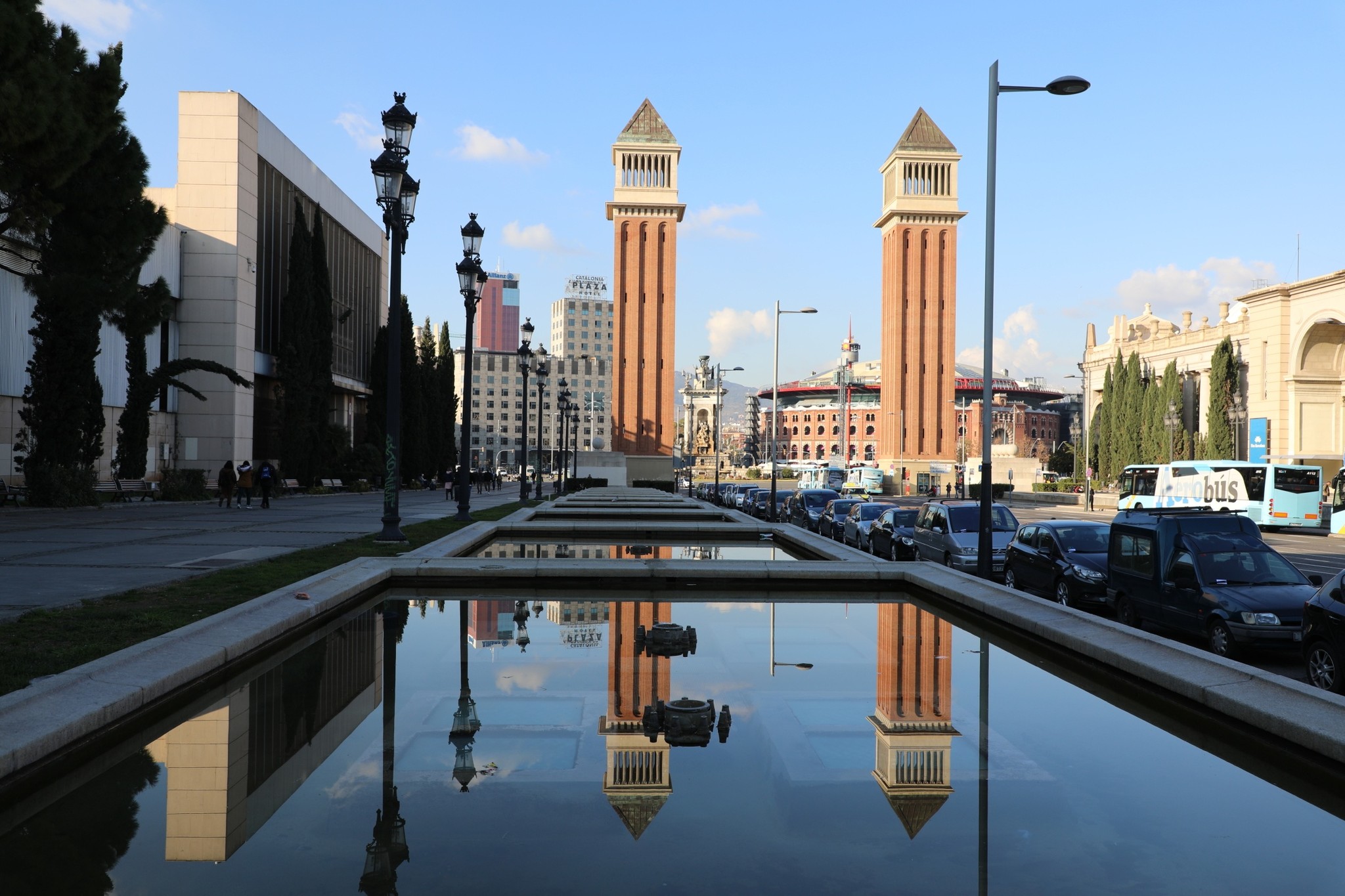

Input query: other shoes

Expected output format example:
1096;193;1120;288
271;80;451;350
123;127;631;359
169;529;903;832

488;492;490;495
260;503;264;508
237;504;241;509
246;504;252;509
451;497;454;500
266;506;269;508
218;501;222;507
227;506;231;508
485;491;487;493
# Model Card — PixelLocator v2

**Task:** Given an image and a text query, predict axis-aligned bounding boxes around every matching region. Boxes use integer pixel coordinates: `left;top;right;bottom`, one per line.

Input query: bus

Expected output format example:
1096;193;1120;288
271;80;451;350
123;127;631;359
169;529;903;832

1118;460;1323;534
1330;467;1345;535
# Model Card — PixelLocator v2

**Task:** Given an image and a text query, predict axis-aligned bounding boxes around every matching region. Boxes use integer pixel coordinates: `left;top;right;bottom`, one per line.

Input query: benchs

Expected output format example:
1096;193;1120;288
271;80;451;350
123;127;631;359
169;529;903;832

359;479;375;491
280;479;308;495
203;479;219;498
0;478;22;508
92;478;156;503
321;479;350;493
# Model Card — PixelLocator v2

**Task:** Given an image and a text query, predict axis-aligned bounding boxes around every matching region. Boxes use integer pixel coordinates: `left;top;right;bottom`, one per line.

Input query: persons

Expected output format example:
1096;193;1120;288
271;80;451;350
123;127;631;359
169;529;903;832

468;468;476;502
1323;481;1331;502
236;461;254;509
1089;487;1095;511
453;467;461;501
697;420;708;438
531;471;536;484
257;459;276;508
477;470;484;494
497;473;502;490
419;474;425;484
955;482;960;498
218;460;237;509
492;470;497;490
946;482;951;497
927;485;940;497
444;466;454;499
484;469;493;494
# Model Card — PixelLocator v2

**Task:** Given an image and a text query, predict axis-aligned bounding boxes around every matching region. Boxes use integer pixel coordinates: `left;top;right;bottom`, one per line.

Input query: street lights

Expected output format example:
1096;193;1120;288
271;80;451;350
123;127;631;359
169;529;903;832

1030;438;1041;458
1164;397;1181;463
534;343;581;501
369;89;418;541
948;397;965;501
713;362;744;507
1227;388;1246;461
358;540;549;896
768;599;816;678
978;60;1092;580
517;316;535;499
886;410;903;498
451;212;491;522
688;395;711;498
769;297;817;523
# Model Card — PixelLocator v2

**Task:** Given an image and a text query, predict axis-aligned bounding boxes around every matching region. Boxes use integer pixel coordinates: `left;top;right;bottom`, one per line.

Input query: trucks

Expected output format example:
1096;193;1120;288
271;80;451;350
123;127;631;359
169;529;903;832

519;465;535;477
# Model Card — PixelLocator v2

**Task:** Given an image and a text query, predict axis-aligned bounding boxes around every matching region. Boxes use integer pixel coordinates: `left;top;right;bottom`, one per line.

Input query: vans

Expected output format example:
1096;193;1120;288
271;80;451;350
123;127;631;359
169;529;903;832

496;469;508;477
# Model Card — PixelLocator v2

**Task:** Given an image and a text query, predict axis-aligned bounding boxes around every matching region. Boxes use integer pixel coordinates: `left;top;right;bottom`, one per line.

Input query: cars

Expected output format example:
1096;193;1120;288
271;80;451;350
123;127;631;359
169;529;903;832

1301;567;1345;697
470;468;477;473
696;466;1151;612
678;478;695;488
541;468;563;475
455;465;460;472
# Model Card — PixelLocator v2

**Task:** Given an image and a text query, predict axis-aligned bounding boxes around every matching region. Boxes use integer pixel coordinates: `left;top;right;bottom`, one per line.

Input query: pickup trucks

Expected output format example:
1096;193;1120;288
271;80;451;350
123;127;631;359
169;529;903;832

1107;505;1323;665
